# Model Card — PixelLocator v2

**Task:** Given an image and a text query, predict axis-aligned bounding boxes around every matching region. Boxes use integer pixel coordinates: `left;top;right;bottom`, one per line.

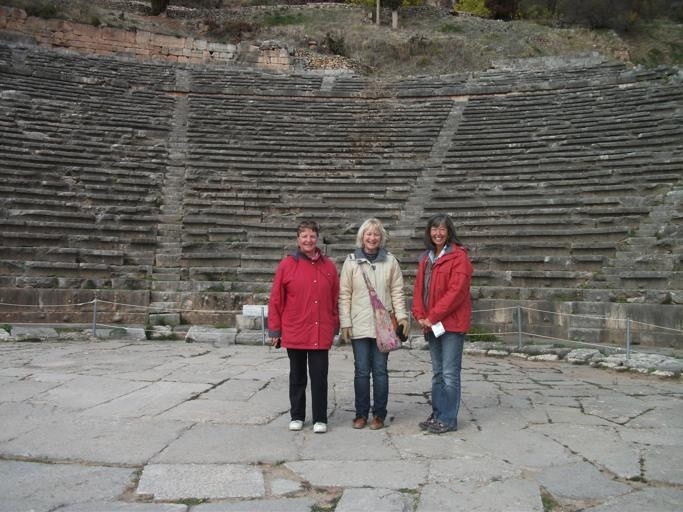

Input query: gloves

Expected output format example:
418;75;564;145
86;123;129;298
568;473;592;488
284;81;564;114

341;327;351;344
399;320;410;338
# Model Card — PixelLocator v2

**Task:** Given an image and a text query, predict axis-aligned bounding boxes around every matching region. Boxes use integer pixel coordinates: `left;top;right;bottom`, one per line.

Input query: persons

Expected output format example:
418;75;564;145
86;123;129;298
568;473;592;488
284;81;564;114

337;218;410;431
412;213;472;435
267;221;339;434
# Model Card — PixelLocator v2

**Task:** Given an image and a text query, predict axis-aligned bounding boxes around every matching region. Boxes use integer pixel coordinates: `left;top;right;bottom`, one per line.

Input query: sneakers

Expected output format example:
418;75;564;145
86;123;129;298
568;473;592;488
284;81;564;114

289;419;327;433
419;416;457;433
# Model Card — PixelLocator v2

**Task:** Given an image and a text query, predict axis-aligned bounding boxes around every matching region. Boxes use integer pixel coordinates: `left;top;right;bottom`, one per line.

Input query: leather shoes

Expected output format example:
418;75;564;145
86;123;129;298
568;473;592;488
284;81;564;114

353;416;384;429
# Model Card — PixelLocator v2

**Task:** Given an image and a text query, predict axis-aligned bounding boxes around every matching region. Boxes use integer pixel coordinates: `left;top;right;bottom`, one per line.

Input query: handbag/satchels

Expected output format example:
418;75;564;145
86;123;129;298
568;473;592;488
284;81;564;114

369;289;401;354
